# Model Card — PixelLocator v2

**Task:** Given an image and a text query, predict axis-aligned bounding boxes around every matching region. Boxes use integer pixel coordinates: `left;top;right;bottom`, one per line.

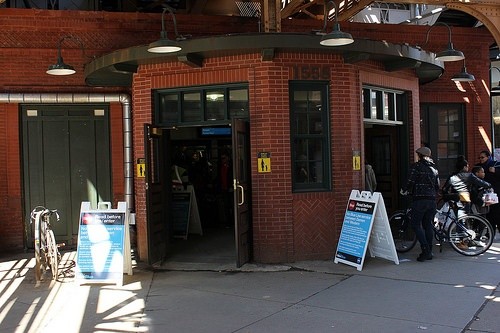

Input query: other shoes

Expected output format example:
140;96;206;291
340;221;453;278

471;240;486;246
457;242;468;250
474;232;482;240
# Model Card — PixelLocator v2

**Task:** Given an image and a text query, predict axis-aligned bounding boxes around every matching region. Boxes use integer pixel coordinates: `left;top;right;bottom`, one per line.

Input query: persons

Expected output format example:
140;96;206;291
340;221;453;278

300;152;308;183
399;146;441;263
215;151;236;229
475;150;500;234
364;160;377;195
467;164;490;241
186;150;218;232
441;155;495;250
493;147;500;167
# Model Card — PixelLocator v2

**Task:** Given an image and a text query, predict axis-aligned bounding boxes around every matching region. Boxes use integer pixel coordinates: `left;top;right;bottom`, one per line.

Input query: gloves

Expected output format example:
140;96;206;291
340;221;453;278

400;189;409;196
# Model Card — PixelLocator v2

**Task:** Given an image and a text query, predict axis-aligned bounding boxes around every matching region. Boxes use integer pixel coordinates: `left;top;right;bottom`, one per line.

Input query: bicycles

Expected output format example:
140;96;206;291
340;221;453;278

388;190;494;256
29;206;66;281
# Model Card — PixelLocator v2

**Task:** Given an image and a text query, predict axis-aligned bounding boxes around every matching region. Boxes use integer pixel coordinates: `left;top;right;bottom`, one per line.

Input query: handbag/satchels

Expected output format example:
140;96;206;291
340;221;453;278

482;187;499;207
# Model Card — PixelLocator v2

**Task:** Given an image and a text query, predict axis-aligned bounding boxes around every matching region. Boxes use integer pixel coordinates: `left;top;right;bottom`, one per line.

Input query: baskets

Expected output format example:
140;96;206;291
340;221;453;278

471;203;490;216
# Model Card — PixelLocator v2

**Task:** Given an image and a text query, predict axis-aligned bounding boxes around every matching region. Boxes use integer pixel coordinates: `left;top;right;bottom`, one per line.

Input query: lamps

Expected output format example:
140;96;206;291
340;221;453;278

46;36;98;75
414;22;464;61
490;81;500;93
147;3;192;53
451;59;475;81
316;0;354;46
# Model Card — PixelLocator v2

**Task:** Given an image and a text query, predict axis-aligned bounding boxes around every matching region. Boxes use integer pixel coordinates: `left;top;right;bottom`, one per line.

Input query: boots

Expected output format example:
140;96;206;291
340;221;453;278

427;244;432;260
417;241;430;261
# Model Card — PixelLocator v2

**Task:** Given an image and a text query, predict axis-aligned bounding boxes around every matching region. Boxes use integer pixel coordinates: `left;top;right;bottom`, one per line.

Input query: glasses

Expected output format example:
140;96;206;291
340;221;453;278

478;156;487;159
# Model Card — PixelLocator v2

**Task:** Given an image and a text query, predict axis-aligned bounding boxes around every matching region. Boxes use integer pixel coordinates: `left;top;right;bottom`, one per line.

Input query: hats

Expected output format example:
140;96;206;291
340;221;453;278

415;147;431;156
